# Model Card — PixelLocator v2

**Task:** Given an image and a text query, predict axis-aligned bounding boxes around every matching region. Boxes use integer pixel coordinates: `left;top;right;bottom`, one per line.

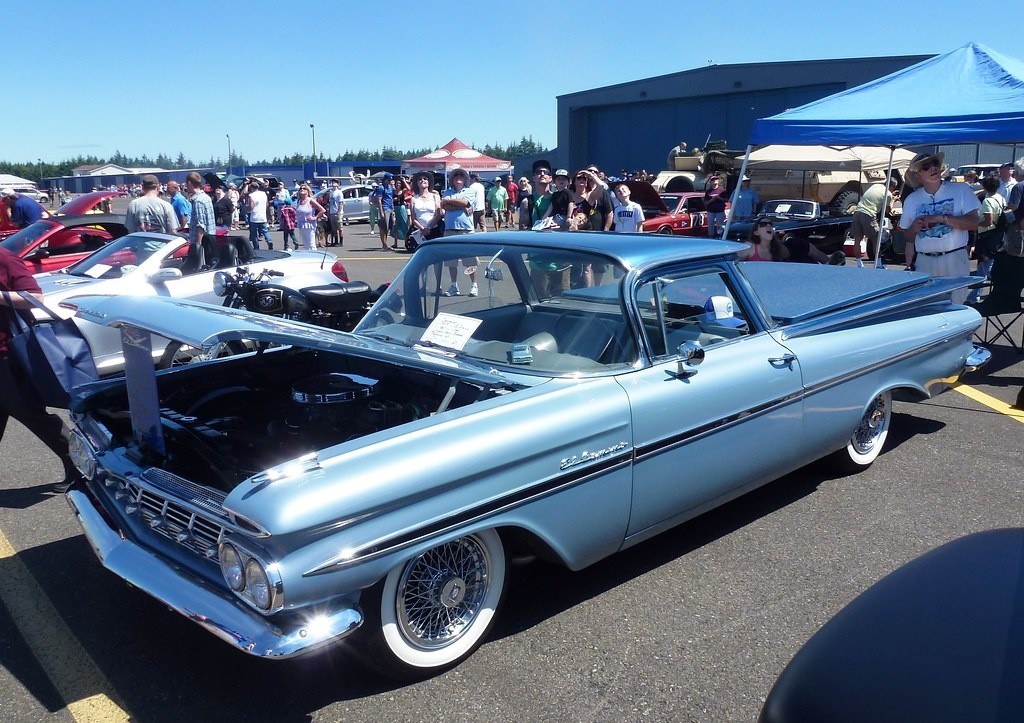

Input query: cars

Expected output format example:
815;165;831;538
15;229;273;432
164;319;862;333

202;171;324;225
0;183;230;276
714;197;854;254
57;231;993;682
475;180;496;217
311;184;374;221
606;179;735;235
14;230;350;378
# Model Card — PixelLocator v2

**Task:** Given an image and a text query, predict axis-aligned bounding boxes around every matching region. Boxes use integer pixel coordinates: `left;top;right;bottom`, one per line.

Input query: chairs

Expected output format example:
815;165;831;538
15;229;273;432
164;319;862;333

962;253;1024;357
604;322;727;364
80;234;108;251
177;243;205;275
201;244;239;272
510;313;616;363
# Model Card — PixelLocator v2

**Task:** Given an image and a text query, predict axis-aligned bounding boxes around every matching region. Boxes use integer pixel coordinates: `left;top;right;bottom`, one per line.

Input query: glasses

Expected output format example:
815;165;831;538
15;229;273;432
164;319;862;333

758;222;775;228
742;180;750;182
588;170;597;174
419;177;427;180
916;159;940;173
454;172;464;177
535;170;550;175
576;177;587;181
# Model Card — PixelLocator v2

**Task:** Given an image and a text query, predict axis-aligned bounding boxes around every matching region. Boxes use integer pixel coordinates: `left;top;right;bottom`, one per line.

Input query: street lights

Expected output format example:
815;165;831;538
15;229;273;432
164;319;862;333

309;124;316;172
226;134;232;173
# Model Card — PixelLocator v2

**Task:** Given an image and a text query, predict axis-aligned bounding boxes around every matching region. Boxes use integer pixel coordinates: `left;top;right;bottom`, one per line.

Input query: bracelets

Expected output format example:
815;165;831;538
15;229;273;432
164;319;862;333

448;198;450;203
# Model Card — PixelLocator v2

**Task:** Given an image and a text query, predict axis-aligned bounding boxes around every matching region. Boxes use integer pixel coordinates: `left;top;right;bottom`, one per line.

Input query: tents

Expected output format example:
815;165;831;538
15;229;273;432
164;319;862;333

400;138;512;189
721;40;1024;268
733;143;918;206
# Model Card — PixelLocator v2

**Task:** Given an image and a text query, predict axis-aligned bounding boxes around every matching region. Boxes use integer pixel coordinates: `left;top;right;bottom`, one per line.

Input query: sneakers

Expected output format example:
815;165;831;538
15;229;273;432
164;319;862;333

444;285;461;297
470;285;478;296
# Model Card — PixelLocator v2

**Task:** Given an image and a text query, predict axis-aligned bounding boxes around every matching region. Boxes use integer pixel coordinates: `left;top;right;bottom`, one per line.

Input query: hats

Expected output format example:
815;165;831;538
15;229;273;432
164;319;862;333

413;170;434;187
1001;162;1014;168
532;160;551;174
742;175;751;180
0;188;15;202
384;174;395;180
228;177;340;205
904;152;945;189
710;175;719;183
451;166;469;181
950;168;957;172
554;168;571;183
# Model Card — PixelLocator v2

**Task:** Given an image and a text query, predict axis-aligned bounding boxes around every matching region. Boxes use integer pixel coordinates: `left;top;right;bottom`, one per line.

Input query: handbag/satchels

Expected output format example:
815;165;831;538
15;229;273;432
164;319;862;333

975;222;1003;252
405;225;424;254
3;291;100;410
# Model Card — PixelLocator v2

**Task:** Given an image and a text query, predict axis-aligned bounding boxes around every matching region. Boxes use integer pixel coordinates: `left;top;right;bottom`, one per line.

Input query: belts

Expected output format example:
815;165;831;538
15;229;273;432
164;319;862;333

920;246;967;256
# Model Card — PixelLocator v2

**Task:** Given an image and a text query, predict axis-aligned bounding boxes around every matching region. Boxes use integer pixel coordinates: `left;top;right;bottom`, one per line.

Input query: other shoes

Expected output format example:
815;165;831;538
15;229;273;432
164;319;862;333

370;228;410;252
420;286;442;297
230;221;343;251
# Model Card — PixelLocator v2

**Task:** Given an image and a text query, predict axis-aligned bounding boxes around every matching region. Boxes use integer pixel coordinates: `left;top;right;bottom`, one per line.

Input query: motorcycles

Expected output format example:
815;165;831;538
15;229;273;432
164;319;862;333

157;257;405;369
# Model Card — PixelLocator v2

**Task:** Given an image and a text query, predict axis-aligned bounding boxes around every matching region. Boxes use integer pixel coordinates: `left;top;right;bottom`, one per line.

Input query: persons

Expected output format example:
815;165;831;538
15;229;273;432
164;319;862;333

48;142;1024;305
1;188;42;229
0;247;76;493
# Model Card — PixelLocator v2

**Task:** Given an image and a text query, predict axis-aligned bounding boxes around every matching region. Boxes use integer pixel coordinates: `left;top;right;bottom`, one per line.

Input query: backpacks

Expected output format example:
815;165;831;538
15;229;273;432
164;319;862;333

988;196;1016;232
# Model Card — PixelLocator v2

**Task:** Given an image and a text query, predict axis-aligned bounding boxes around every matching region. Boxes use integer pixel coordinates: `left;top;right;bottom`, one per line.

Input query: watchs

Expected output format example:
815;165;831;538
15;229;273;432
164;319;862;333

942;213;949;224
